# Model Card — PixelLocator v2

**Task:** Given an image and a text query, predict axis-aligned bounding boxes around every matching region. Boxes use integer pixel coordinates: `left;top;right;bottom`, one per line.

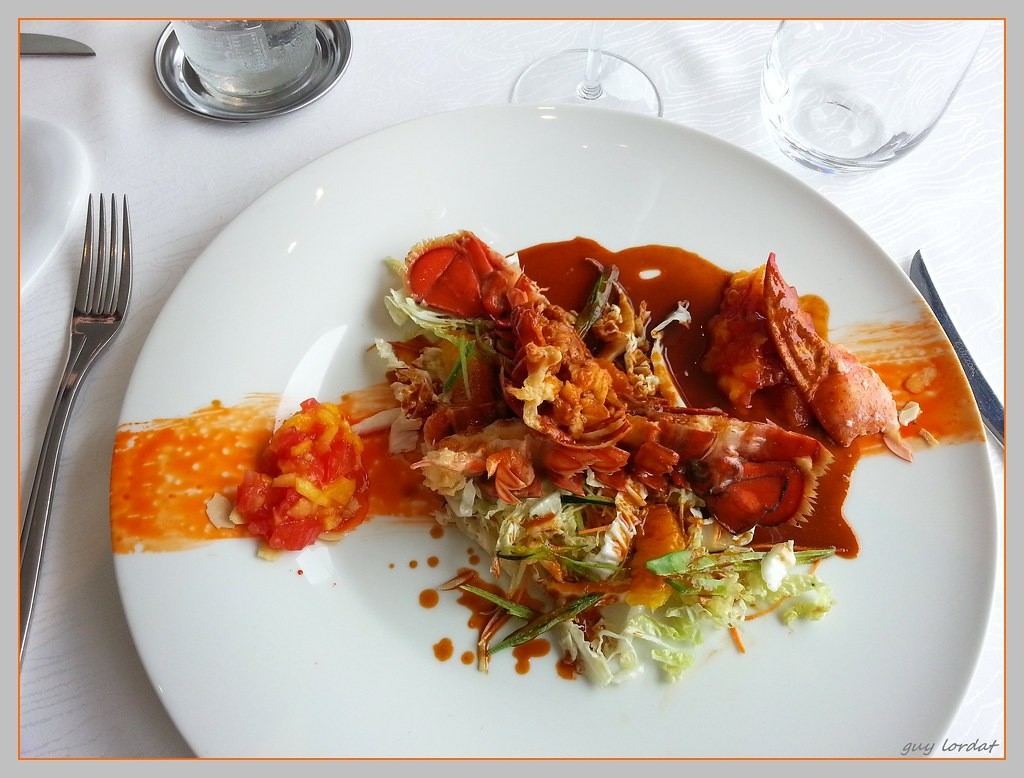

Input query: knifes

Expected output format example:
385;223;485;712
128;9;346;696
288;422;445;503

908;248;1005;453
19;32;97;58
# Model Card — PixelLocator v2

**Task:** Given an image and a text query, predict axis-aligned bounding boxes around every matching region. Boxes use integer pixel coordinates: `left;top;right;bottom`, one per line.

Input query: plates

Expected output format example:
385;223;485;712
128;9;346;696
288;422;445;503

150;21;354;125
108;103;1001;759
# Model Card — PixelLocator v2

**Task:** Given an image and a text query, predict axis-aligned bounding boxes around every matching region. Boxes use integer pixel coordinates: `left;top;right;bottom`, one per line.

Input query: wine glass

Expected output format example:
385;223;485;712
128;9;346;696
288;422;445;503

491;20;663;117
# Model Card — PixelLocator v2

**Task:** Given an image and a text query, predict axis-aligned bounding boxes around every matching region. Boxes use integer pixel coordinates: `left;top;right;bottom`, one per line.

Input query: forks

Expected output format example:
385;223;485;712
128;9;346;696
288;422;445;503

18;192;136;665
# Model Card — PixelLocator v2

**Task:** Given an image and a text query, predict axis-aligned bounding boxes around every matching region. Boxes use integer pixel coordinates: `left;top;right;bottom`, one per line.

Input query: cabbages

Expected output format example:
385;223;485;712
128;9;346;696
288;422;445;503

352;287;830;689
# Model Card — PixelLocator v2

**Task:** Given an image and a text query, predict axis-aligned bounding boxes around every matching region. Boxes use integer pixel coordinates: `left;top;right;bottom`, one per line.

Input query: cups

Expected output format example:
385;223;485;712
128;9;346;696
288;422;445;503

755;19;991;175
162;18;321;106
